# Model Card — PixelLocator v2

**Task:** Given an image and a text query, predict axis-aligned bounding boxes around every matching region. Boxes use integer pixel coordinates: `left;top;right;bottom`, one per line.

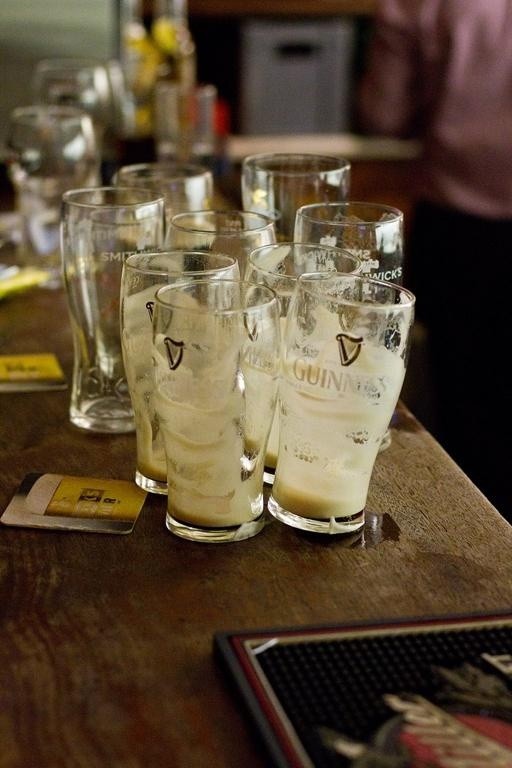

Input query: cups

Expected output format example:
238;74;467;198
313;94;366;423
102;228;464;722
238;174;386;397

119;251;240;495
153;80;214;169
11;52;118;267
153;279;282;544
294;200;404;454
63;187;169;433
164;208;278;281
115;163;213;249
241;241;361;487
242;153;351;245
268;271;415;534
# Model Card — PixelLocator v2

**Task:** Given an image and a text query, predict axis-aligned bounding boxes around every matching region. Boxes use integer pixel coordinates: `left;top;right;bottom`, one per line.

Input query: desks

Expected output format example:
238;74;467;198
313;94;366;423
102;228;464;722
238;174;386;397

2;172;509;767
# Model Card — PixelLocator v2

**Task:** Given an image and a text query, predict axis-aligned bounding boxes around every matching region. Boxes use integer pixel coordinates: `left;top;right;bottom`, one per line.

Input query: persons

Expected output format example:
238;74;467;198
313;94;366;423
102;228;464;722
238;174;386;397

350;2;512;530
